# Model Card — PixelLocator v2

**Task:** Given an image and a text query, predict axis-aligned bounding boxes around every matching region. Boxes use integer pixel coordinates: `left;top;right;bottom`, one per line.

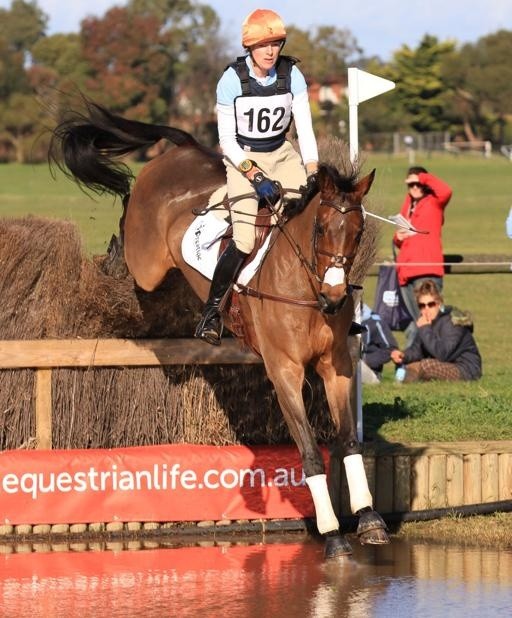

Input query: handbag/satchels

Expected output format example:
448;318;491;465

373;263;413;332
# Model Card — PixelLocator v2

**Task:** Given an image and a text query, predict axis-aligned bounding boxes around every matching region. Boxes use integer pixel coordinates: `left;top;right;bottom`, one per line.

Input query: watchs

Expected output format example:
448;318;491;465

239;159;258;175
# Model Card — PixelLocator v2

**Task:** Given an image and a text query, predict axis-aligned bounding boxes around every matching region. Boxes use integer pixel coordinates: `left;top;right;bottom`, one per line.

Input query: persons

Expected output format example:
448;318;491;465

393;165;453;291
354;300;399;383
388;280;483;384
192;7;322;350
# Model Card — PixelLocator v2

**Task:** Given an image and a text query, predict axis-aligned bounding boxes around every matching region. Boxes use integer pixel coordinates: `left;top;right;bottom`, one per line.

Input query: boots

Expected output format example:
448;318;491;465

194;238;250;346
349;323;368;336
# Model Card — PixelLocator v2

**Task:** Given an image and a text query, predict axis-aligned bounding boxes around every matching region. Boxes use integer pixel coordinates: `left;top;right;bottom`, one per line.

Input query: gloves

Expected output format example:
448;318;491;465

249;172;280;203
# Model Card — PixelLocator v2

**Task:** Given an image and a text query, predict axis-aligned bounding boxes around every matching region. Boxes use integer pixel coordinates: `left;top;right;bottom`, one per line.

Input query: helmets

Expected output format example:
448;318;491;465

242;8;287;48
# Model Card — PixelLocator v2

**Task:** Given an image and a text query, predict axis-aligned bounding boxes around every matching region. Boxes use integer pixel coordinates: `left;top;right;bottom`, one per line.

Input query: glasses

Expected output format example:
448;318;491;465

418;302;436;310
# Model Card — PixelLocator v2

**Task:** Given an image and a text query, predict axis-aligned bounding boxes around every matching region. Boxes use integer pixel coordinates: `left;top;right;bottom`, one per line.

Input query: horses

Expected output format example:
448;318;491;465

29;79;392;562
